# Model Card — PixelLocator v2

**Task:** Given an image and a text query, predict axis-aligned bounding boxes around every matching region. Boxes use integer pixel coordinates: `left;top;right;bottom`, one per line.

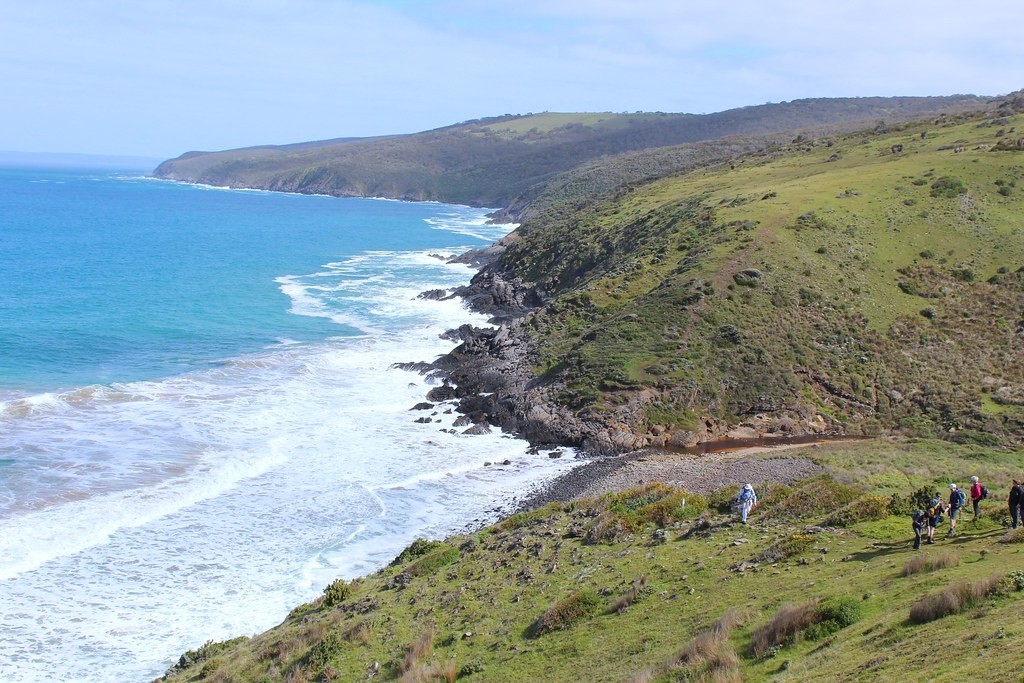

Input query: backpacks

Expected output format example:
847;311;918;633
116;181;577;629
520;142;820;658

930;499;939;509
955;490;966;505
980;485;989;501
912;510;922;520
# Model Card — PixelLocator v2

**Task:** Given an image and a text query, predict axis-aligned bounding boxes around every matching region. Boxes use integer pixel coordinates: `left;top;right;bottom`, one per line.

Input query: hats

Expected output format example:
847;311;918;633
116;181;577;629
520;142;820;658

1013;479;1021;484
949;484;956;489
745;484;752;489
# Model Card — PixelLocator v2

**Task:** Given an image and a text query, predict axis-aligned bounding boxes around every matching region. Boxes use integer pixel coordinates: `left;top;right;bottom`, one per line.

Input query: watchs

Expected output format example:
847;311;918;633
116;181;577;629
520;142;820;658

947;505;950;509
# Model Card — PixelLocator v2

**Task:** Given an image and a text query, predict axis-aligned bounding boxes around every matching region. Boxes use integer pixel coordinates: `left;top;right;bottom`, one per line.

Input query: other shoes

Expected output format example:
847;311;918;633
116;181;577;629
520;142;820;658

948;531;953;536
928;540;935;544
742;521;746;524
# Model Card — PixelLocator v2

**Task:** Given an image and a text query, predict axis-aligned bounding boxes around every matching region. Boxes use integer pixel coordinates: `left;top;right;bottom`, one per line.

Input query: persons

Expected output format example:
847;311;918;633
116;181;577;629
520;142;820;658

926;508;935;544
945;483;965;537
737;483;758;525
970;476;981;522
1008;479;1024;529
912;510;925;550
926;491;952;544
